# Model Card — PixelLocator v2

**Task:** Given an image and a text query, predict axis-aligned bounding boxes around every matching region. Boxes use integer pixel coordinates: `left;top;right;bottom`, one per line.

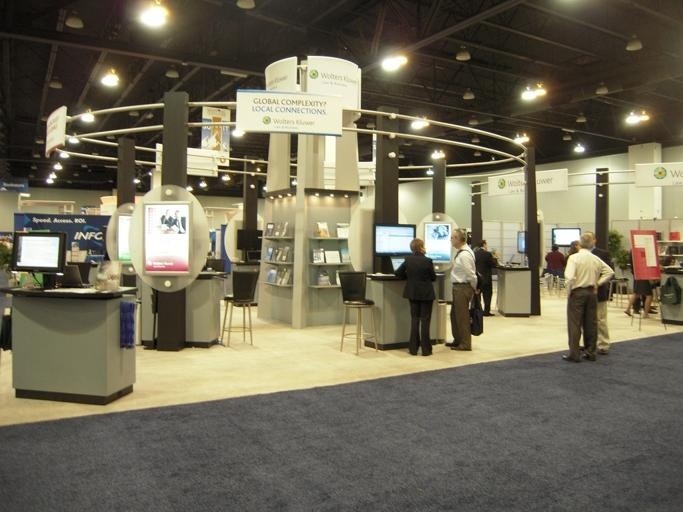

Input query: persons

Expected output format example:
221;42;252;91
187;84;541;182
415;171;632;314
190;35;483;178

445;227;481;351
394;238;437;356
567;240;579;255
470;239;495;317
544;243;565;274
560;233;616;363
579;230;613;355
624;247;658;318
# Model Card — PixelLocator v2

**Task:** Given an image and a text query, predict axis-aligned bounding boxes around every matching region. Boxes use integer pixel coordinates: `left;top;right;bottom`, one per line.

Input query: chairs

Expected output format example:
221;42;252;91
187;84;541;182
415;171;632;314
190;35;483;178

337;271;379;354
221;269;259;346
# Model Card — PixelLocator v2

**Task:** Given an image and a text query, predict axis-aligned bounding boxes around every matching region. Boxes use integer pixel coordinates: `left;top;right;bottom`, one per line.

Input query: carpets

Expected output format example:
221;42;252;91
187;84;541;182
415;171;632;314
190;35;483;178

0;331;682;512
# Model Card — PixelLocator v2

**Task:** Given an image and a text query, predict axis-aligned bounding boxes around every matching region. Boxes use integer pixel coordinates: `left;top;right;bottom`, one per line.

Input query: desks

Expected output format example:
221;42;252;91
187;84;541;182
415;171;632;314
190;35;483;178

658;273;683;325
141;273;227;348
0;286;140;405
497;267;532;317
363;273;445;349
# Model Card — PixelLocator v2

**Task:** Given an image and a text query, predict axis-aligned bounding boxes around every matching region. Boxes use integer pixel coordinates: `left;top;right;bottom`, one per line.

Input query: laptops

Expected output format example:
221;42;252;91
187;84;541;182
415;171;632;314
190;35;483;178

62;265;92;288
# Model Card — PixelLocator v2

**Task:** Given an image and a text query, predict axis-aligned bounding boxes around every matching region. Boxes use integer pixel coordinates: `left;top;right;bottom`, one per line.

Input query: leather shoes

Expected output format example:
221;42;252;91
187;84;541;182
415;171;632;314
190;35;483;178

445;341;457;347
450;345;469;351
484;312;495;316
562;346;608;362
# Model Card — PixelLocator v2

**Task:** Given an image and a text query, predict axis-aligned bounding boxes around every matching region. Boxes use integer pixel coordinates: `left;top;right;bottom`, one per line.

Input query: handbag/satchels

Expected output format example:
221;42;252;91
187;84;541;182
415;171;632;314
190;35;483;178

454;250;485;293
469;294;483;336
660;276;682;306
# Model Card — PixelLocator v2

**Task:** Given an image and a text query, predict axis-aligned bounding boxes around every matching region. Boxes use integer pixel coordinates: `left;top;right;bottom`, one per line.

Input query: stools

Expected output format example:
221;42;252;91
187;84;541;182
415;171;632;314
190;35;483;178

546;273;630;310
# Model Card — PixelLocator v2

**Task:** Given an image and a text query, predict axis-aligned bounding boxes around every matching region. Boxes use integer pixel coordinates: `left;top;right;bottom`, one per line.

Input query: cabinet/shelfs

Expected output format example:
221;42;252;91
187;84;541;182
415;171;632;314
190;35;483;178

308;237;348;289
258;236;293;288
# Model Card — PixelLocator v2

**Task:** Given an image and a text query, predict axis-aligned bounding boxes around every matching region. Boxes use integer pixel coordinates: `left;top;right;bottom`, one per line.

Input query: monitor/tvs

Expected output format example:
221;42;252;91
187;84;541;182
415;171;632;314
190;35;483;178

373;223;416;257
517;231;526;253
11;231;67;273
552;228;581;247
